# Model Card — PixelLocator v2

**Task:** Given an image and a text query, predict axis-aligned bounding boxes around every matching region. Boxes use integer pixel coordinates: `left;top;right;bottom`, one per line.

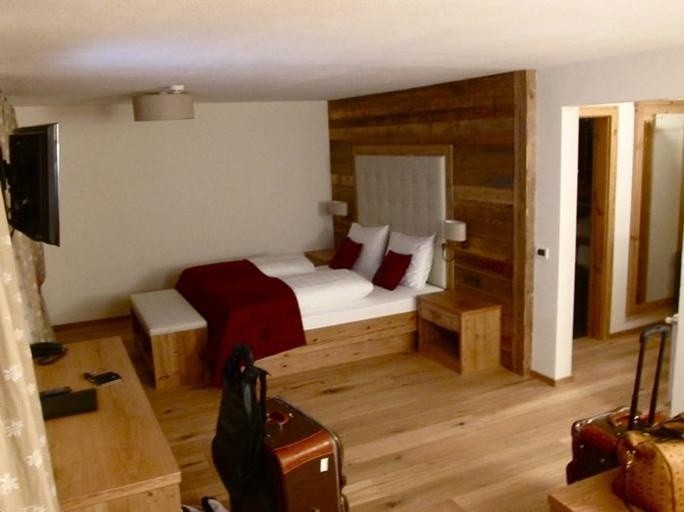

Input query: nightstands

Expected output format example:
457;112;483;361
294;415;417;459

416;291;503;376
304;249;337;266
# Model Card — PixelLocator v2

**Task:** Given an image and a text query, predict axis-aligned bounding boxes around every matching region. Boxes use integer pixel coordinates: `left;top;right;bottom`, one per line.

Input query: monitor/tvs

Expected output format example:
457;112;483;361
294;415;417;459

7;122;61;247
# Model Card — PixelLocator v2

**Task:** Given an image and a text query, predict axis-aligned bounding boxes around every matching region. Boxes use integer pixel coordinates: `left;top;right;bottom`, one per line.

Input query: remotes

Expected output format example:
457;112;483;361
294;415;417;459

38;386;70;398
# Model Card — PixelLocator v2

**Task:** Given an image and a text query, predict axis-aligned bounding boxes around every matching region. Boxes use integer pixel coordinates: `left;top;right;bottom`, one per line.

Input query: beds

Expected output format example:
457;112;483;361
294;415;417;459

175;145;454;390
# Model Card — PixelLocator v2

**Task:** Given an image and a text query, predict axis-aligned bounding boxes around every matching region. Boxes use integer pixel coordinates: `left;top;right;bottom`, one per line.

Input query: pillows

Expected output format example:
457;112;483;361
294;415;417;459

371;250;413;291
347;222;389;282
328;236;362;269
384;231;436;291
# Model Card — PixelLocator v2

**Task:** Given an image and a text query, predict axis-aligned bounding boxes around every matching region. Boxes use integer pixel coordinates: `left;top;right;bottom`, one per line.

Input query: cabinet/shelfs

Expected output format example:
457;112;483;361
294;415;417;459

33;336;182;511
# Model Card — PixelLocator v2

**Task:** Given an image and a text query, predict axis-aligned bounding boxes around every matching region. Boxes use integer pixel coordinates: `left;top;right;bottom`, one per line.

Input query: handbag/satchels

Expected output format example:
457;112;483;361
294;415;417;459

613;412;682;512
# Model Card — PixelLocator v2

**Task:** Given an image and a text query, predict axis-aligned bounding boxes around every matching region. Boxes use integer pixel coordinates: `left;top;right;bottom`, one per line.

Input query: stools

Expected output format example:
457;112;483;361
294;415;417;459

127;289;208;393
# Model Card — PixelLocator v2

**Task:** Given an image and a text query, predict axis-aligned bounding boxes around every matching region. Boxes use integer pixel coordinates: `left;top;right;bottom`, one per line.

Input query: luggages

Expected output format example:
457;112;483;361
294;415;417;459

567;324;670;486
212;357;349;512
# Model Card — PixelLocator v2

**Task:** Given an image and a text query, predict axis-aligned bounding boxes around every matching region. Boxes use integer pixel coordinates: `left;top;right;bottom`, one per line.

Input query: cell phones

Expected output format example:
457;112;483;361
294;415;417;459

92;372;119;384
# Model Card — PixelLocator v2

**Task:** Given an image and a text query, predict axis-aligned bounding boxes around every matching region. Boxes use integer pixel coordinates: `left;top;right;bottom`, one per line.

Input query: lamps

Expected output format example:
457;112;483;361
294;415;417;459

328;200;347;216
441;220;466;262
133;90;195;122
